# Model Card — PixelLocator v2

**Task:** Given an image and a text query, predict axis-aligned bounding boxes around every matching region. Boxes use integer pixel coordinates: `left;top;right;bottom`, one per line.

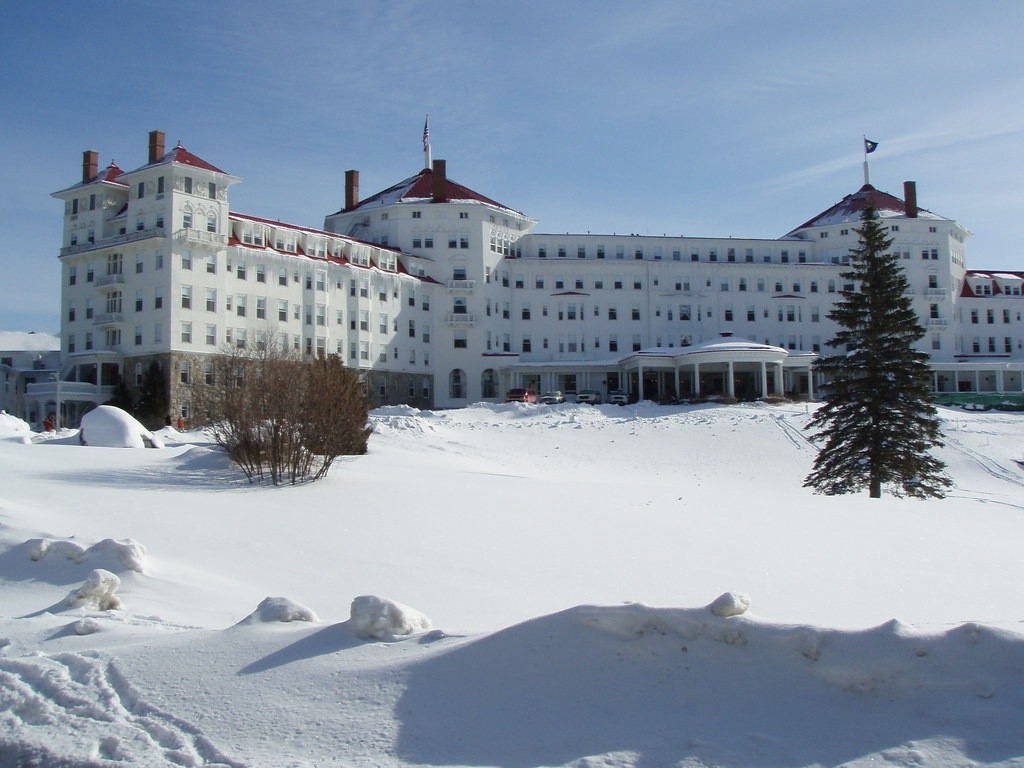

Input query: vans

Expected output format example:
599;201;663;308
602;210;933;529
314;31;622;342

576;389;602;404
506;387;537;404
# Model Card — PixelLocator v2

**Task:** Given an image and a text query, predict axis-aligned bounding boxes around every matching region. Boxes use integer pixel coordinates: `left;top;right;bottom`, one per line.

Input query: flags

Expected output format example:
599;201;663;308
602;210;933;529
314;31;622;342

423;120;429;154
865;139;878;153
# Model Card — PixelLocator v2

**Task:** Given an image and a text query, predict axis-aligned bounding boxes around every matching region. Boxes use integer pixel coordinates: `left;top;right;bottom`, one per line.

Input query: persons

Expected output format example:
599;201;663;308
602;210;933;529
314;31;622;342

43;418;51;431
178;415;185;433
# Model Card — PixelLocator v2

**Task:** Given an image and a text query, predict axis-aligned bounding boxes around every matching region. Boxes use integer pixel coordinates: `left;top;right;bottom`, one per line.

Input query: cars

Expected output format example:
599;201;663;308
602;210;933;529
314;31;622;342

608;391;628;403
539;391;564;404
